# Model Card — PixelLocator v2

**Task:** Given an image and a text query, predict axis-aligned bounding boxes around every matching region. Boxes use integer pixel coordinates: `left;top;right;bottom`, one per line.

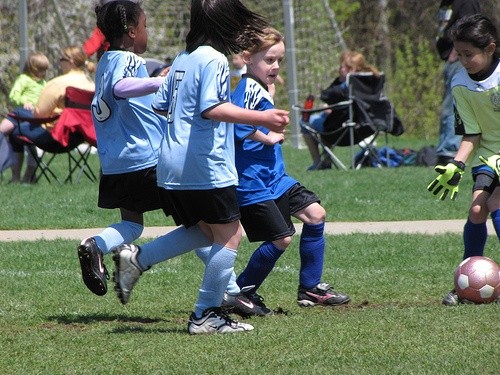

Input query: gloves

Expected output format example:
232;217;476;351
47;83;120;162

427;159;465;202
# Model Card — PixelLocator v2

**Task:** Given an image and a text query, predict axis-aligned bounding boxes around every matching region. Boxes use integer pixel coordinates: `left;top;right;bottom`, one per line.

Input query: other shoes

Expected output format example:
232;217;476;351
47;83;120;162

9;176;40;185
307;162;332;171
442;289;460;306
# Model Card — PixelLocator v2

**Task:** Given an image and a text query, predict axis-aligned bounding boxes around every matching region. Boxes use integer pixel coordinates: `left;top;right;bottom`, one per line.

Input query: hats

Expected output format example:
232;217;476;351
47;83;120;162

83;29;110;56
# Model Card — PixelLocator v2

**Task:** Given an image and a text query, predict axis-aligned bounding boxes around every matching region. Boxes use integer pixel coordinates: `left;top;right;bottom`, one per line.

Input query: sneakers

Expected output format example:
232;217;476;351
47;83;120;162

297;282;352;309
112;243;143;305
187;306;254;336
78;237;110;296
222;296;265;317
243;292;271;314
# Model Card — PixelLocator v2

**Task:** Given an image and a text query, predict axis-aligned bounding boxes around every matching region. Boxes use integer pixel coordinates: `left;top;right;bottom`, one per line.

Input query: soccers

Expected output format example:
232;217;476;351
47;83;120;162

455;256;500;305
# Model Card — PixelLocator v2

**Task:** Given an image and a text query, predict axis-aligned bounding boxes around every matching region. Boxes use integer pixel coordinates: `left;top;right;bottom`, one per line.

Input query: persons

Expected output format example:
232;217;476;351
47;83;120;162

0;0;351;335
298;50;380;172
427;0;500;306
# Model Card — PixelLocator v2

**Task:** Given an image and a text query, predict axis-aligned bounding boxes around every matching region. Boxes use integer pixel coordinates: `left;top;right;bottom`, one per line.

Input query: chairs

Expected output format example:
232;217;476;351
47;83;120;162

1;86;98;185
292;71;405;171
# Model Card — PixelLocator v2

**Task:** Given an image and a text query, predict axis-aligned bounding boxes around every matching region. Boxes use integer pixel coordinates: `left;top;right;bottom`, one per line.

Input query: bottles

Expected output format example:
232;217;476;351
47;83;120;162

302;95;314;121
